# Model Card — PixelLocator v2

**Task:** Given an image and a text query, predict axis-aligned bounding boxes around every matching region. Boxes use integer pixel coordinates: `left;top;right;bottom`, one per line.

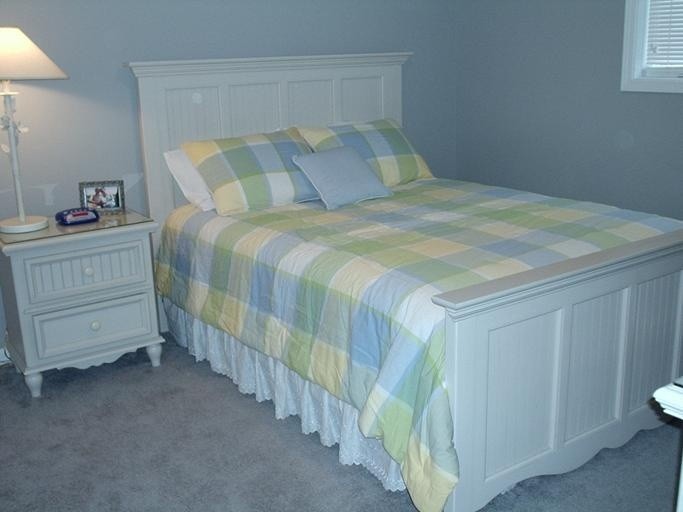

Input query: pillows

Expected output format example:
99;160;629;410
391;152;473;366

296;116;433;191
163;149;216;212
292;145;394;211
180;126;322;216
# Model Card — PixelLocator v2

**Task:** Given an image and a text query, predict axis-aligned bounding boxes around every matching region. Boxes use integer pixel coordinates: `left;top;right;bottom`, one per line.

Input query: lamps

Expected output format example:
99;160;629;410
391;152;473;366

0;26;70;235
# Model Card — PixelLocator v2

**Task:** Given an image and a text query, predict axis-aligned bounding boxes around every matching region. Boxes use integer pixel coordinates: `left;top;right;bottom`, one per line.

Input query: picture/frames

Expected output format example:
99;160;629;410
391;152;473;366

79;179;126;215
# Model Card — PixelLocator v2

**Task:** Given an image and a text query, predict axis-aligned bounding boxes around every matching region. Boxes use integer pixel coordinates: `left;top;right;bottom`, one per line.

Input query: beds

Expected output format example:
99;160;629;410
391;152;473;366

128;51;682;512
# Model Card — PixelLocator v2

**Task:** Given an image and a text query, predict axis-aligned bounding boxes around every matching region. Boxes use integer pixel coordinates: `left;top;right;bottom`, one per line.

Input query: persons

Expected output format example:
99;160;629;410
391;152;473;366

88;187;116;208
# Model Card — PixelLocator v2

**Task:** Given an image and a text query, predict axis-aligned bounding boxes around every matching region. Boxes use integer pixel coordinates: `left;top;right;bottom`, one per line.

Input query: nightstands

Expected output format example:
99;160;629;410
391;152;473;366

0;209;166;398
